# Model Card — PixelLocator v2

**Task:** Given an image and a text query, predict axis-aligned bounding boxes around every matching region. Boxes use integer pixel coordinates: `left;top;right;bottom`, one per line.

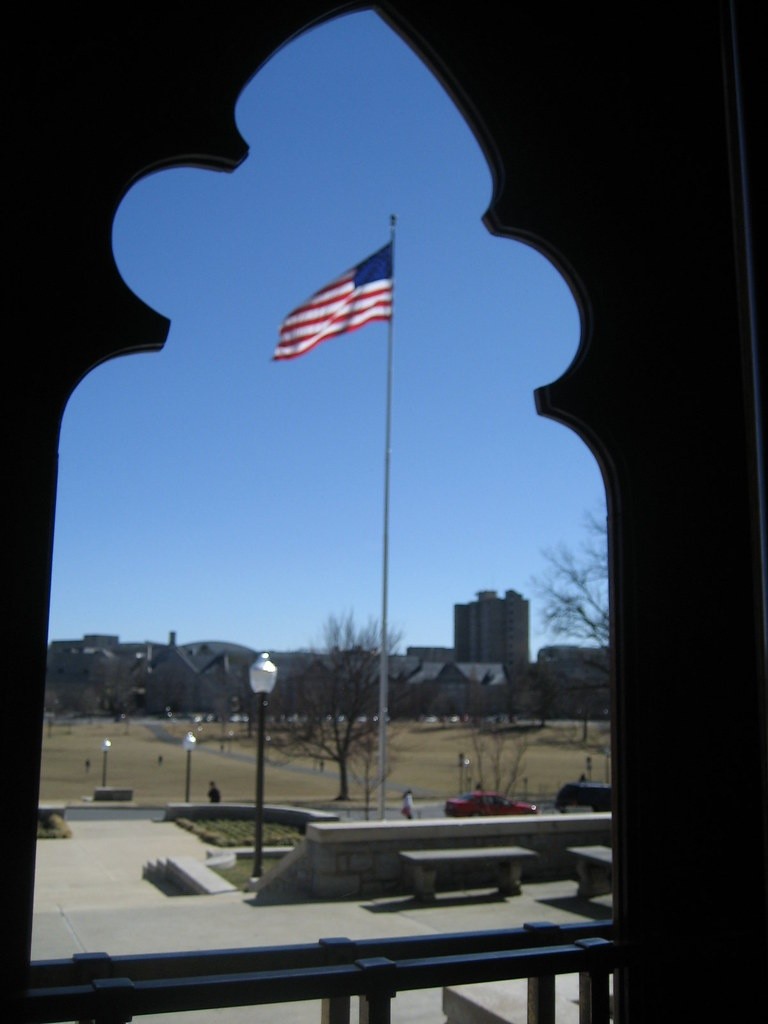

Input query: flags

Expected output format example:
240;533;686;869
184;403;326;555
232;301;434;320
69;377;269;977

268;236;392;361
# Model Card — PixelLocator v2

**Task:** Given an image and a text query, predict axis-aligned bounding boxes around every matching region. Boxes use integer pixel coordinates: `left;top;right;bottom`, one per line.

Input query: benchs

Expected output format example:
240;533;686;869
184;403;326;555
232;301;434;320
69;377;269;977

82;789;133;802
568;841;615;896
399;845;540;905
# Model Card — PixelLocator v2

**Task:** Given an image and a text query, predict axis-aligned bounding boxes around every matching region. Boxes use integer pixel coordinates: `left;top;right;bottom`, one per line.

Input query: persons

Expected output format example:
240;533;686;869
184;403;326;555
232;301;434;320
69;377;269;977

207;781;220;803
400;790;416;819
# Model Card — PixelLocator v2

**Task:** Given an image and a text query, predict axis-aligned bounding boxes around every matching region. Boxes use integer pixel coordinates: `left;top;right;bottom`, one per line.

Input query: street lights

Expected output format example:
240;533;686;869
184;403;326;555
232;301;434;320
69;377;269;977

183;731;197;804
248;651;278;894
101;738;112;787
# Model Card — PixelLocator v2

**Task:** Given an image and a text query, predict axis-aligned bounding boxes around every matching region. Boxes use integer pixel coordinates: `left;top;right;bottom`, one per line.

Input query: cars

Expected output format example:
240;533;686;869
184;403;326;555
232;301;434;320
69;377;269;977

553;783;613;815
443;792;540;817
